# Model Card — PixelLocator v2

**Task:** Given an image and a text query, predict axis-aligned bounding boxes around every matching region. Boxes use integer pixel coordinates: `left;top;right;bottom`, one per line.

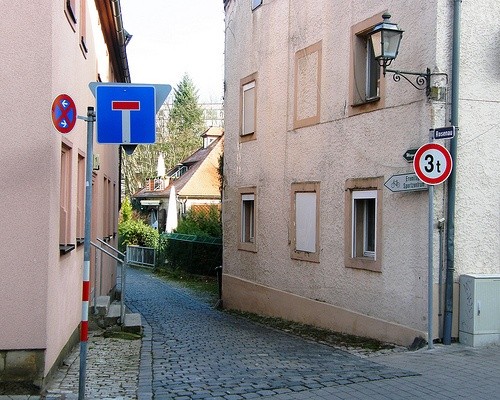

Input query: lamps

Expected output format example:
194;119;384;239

366;12;430;95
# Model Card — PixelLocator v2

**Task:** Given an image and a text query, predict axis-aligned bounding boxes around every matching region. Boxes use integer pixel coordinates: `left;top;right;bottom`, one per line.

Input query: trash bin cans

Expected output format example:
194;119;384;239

215;266;222;298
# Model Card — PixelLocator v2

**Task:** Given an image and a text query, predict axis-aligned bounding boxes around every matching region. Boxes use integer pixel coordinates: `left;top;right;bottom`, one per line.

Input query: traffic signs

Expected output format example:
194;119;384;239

403;147;420;163
434;126;456;140
383;172;428;192
